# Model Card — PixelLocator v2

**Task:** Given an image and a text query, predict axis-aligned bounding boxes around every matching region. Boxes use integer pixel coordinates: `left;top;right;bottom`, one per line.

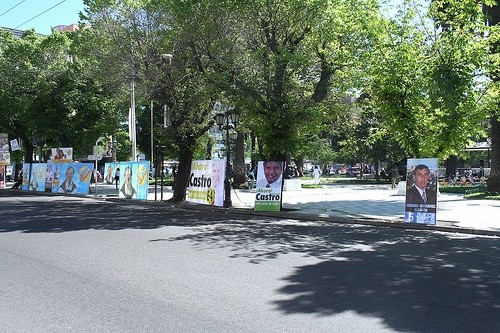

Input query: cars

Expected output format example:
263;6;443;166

346;167;362;178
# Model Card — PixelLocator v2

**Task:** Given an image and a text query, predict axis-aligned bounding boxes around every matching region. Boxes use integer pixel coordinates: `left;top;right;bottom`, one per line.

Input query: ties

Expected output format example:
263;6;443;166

422;192;427;204
265;184;271;187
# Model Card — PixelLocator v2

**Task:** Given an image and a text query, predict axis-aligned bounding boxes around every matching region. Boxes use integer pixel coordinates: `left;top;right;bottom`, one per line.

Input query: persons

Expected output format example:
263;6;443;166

284;165;303;179
448;167;484;186
311;164;331;185
406;164;436;212
121;167;136;199
165;166;178;175
105;166;114;184
24;165;45;191
57;166;78;194
230;167;257;189
11;171;23;189
115;167;120;190
46;148;65;186
382;165;398;188
263;161;282;188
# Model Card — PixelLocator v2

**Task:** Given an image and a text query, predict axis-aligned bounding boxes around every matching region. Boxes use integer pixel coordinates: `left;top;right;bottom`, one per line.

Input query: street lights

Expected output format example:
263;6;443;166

162;53;175;146
